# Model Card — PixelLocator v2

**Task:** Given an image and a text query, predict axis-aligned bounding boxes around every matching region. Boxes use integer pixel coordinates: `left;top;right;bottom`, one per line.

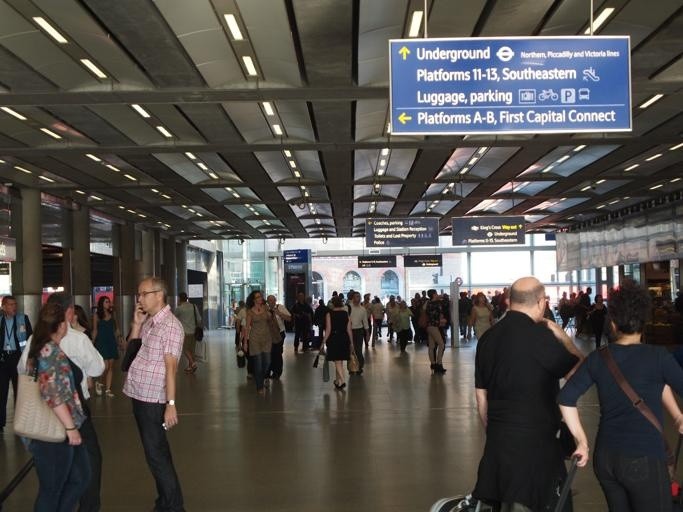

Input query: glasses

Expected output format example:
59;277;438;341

135;290;159;298
537;296;550;304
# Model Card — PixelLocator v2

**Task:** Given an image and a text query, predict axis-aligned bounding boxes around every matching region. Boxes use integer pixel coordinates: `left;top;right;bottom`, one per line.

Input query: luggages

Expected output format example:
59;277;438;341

428;455;578;512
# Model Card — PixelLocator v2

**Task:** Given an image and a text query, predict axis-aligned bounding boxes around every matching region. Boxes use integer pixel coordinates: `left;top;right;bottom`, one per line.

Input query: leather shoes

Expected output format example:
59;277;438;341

94;382;103;395
104;389;114;397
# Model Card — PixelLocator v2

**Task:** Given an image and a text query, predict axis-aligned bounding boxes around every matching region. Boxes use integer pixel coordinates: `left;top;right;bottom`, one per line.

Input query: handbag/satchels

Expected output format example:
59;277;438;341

194;326;204;341
12;358;66;443
348;350;360;372
237;336;245;368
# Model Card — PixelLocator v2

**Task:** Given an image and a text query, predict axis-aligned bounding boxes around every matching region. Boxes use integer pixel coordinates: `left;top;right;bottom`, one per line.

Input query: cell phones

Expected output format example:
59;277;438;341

140;308;146;316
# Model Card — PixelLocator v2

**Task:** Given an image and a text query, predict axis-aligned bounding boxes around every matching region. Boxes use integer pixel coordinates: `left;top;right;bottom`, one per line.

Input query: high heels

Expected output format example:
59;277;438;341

431;364;446;372
334;380;337;387
336;383;345;390
436;363;444;373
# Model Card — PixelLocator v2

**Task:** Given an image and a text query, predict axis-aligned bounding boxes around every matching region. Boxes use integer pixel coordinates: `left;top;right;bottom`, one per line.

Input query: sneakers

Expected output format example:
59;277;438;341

191;364;197;372
184;367;192;372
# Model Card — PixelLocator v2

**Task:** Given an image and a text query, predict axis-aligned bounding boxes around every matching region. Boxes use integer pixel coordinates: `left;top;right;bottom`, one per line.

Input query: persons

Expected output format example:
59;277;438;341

555;279;683;511
471;275;585;512
